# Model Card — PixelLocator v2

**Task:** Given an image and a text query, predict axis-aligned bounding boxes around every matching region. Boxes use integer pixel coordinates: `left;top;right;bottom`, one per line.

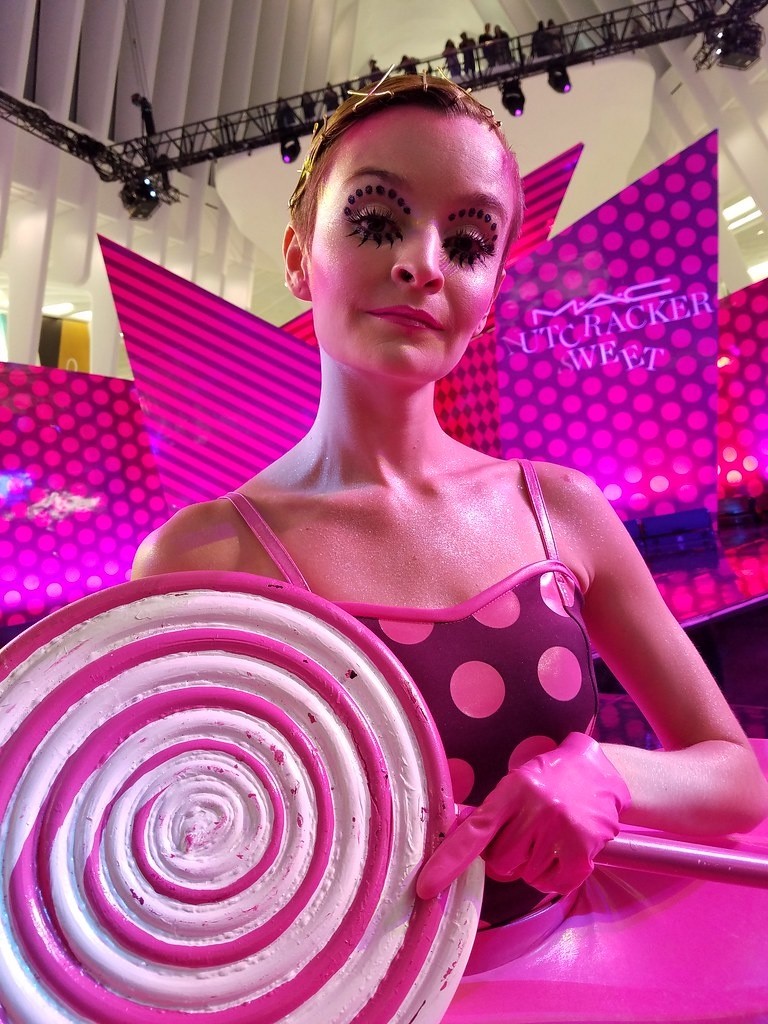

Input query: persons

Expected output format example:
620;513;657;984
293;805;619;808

459;32;477;74
274;98;296;128
323;82;340;112
369;60;384;81
442;39;461;77
530;19;563;57
301;93;315;120
344;78;367;101
130;76;767;1024
396;56;420;75
479;23;514;67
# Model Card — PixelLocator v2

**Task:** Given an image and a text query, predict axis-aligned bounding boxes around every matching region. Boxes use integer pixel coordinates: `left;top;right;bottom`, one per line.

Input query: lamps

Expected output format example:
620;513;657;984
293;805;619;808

548;66;571;92
502;83;525;116
280;138;301;164
